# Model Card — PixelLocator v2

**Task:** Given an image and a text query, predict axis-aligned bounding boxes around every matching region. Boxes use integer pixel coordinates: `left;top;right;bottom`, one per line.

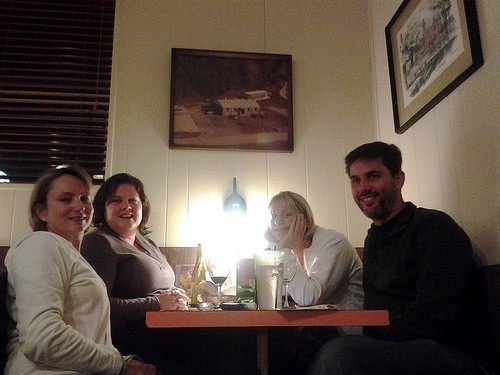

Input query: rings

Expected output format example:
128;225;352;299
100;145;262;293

295;229;299;233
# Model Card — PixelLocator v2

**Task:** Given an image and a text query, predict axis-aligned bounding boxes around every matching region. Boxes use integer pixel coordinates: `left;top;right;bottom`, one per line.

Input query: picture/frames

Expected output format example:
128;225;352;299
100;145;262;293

169;47;293;153
384;0;484;134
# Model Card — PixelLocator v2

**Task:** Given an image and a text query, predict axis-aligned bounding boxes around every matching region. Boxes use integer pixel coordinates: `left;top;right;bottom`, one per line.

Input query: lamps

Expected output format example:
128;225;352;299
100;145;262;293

223;178;247;215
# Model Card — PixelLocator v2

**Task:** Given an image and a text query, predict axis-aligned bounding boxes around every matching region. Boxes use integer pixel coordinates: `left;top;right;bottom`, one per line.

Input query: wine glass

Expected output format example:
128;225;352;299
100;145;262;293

206;259;231;310
281;252;298;310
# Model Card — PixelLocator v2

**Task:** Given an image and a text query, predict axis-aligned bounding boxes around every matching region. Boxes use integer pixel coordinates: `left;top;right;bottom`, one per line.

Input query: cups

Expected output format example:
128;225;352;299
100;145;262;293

255;250;285;312
235;257;257;311
175;264;195;307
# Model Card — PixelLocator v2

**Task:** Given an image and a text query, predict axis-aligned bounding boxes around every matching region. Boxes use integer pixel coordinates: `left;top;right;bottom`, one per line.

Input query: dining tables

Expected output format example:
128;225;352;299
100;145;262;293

146;311;389;375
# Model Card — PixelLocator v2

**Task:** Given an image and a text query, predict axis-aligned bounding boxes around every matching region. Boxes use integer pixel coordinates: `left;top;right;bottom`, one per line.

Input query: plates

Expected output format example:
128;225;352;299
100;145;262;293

220;302;246;310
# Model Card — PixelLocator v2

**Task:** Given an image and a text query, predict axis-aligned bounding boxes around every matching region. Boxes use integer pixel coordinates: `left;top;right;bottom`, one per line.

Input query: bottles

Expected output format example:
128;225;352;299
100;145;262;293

190;242;207;307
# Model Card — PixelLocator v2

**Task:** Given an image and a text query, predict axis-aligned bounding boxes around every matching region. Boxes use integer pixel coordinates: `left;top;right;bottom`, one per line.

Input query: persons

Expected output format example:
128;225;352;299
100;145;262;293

345;142;485;375
261;190;365;375
3;164;191;375
